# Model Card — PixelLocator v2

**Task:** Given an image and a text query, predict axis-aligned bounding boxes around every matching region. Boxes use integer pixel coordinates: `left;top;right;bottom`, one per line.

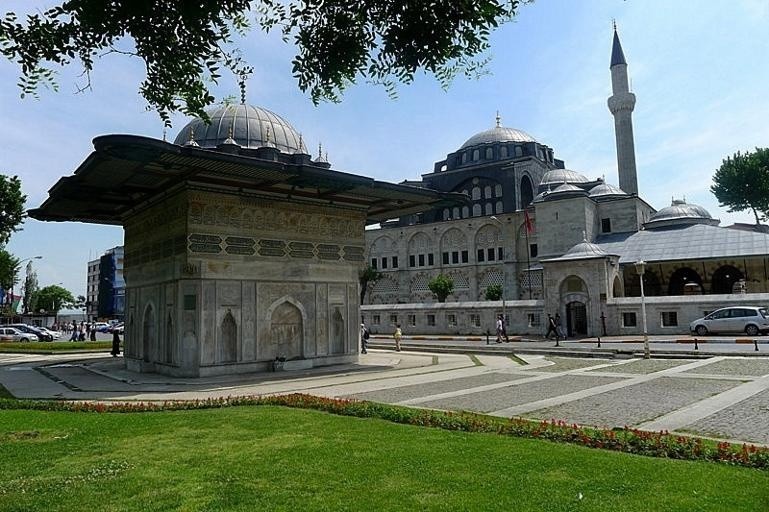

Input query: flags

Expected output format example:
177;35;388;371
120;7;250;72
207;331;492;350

525;209;533;232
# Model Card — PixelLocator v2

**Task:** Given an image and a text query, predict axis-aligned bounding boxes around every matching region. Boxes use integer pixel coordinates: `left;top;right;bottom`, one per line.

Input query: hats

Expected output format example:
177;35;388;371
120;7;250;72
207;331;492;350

360;324;364;326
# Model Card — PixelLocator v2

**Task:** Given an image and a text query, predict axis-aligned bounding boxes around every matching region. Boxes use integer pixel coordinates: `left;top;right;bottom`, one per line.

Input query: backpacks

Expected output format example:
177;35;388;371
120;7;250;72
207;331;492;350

364;329;369;339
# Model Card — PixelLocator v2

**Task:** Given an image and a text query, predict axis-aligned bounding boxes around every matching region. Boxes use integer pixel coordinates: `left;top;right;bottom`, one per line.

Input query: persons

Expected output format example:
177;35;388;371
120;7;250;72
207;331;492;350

495;316;509;343
542;313;567;340
52;320;96;342
393;325;401;351
360;324;369;354
111;330;120;357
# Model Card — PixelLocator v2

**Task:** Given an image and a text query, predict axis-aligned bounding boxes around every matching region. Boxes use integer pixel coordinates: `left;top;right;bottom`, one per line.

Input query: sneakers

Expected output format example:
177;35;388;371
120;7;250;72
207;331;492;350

361;352;367;354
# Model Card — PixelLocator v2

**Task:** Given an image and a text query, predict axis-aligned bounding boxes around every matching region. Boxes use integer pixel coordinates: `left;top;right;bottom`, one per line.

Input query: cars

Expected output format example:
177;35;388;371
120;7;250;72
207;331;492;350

0;323;61;343
689;306;769;336
68;319;124;334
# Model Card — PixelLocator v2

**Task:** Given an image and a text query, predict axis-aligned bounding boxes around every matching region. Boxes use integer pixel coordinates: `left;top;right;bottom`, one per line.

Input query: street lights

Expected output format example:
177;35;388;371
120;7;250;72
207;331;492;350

634;261;650;358
11;256;42;323
515;209;532;299
490;216;506;320
